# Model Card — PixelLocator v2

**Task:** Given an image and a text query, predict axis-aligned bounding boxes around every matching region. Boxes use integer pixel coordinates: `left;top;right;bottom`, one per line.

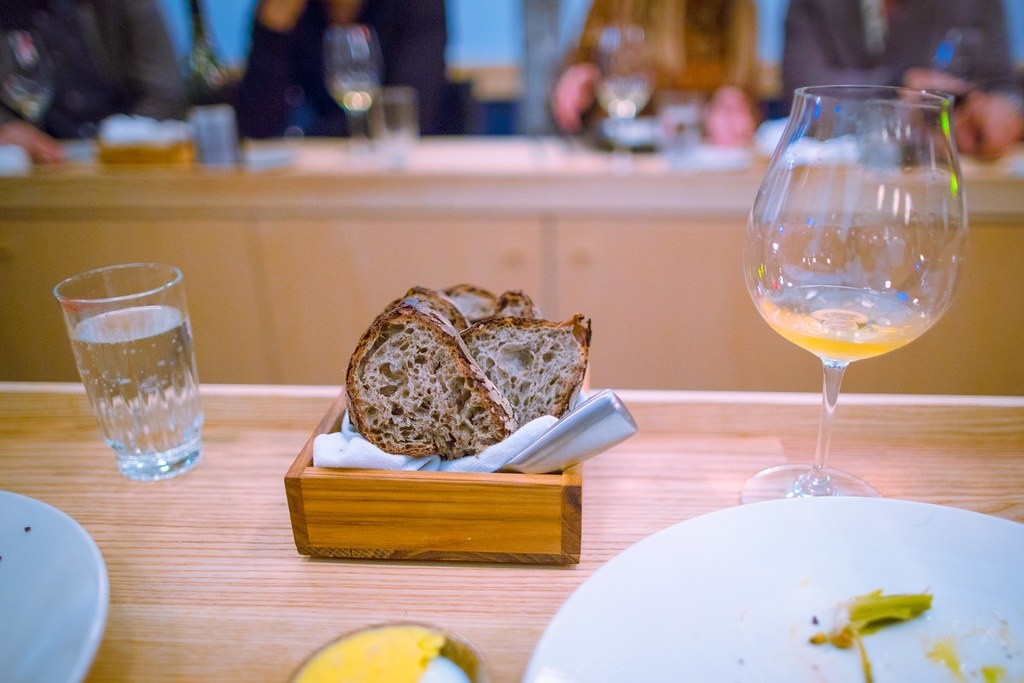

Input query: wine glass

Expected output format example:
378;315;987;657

741;84;968;502
321;25;382;159
588;27;654;168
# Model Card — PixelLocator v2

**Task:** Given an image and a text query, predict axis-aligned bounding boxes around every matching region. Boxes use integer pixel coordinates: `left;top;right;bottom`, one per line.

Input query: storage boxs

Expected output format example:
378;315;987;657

284;403;584;566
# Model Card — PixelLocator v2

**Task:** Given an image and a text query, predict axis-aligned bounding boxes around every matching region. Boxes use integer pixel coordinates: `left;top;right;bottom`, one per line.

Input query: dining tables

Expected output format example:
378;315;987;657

0;132;1024;403
0;380;1024;683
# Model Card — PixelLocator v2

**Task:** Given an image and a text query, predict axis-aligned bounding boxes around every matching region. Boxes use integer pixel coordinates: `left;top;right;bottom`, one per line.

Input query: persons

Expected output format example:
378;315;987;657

235;0;451;142
0;0;180;168
550;0;767;145
784;1;1024;162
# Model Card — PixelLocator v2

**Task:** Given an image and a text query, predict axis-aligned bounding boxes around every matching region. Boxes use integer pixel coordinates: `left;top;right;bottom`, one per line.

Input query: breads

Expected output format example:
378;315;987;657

344;281;592;461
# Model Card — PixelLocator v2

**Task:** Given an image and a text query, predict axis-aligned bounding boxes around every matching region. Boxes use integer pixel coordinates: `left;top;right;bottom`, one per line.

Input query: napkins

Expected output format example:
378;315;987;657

311;414;553;479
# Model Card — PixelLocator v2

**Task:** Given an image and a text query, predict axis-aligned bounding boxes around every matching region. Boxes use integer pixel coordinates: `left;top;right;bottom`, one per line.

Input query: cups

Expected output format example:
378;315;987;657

660;94;702;167
55;262;204;483
365;87;420;153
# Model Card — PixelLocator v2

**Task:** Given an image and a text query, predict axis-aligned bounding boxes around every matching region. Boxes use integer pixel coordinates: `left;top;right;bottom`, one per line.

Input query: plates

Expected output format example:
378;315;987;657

525;497;1024;682
1;488;109;683
